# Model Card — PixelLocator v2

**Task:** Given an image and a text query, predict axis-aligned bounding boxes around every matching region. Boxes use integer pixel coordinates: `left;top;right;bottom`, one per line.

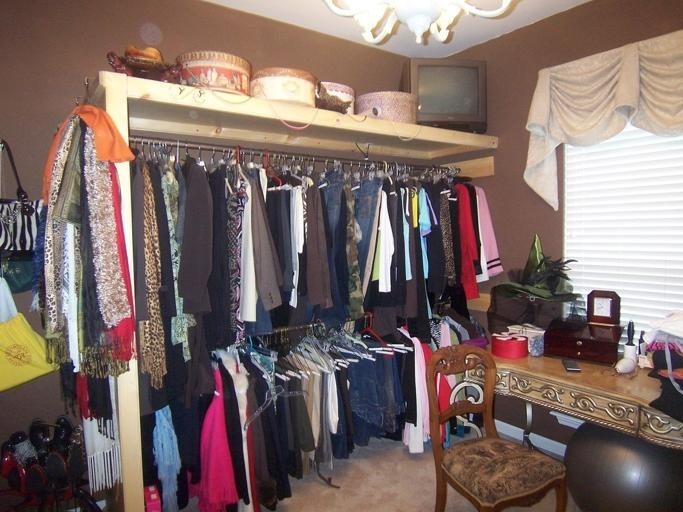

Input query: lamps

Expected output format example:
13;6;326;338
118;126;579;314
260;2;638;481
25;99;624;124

328;0;512;43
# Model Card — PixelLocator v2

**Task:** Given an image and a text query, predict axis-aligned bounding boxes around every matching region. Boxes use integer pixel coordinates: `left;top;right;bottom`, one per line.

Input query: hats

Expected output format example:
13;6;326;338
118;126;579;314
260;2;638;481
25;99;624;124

120;46;170;70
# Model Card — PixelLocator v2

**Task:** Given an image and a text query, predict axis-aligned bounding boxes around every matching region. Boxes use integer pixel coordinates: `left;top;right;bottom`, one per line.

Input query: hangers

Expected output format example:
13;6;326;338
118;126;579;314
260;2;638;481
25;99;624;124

226;300;454;386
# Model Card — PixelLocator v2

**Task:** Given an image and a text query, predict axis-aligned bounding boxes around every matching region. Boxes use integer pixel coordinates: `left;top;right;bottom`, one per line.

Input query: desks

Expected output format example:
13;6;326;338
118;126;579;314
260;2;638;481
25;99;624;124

458;334;682;430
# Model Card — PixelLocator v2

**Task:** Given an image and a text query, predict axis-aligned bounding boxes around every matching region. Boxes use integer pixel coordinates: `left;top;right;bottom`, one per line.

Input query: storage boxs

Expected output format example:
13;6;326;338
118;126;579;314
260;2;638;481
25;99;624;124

179;47;417;124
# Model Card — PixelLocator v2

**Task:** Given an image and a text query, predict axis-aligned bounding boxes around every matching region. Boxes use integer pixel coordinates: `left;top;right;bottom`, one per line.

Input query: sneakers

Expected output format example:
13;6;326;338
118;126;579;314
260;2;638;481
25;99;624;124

0;414;85;505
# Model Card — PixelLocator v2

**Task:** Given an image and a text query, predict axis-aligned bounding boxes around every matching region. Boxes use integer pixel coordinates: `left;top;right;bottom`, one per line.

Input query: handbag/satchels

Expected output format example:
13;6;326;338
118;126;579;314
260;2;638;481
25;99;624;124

0;139;43;294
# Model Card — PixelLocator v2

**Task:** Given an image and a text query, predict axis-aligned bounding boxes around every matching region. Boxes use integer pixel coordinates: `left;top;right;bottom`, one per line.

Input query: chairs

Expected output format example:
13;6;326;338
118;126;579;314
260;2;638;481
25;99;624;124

426;345;567;511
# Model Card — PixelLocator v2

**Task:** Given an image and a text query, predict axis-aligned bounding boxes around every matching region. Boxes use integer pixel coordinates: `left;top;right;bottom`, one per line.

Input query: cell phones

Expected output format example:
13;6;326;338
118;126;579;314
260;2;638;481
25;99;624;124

563;358;581;371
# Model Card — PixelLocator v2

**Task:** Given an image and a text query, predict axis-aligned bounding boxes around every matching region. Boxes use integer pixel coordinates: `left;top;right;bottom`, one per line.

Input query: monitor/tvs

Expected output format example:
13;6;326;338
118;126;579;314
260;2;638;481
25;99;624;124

398;58;487;134
